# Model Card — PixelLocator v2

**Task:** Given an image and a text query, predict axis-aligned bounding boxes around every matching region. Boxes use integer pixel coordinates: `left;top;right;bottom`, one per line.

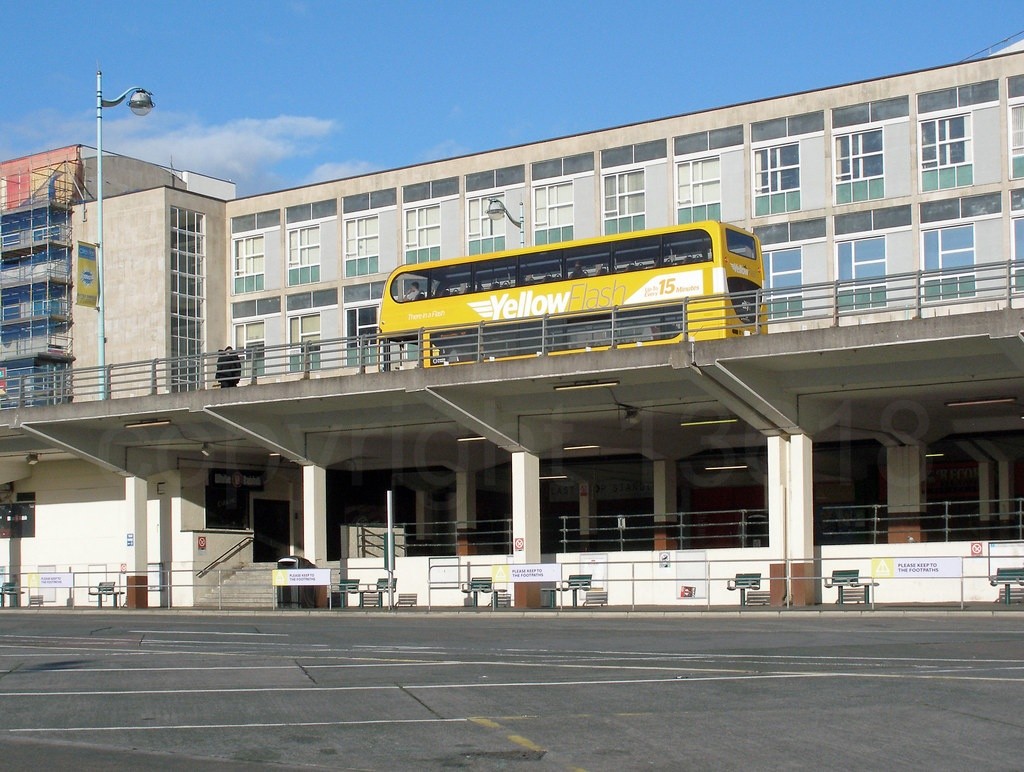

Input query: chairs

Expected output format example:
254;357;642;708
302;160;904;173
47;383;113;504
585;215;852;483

413;251;706;300
995;565;1024;604
0;582;18;607
89;582;125;608
462;576;508;608
540;574;594;607
832;569;878;604
330;576;397;609
727;572;762;606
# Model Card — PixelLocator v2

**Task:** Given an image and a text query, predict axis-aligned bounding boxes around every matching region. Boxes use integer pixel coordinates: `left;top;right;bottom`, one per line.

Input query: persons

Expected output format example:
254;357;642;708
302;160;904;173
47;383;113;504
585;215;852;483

215;346;241;387
404;282;420;301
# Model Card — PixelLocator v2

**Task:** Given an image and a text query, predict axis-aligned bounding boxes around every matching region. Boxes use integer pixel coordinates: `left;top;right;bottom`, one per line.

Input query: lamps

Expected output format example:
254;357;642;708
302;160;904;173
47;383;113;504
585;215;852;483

704;464;749;472
554;380;620;392
625;410;642;426
201;443;214;457
944;396;1019;408
563;445;600;451
456;435;486;442
124;419;170;429
25;452;38;465
539;475;567;480
681;417;739;427
926;452;944;458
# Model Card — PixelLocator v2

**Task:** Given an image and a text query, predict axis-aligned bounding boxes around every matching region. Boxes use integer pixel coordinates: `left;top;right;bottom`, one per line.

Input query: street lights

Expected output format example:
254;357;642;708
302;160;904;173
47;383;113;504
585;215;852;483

94;69;157;400
484;197;525;248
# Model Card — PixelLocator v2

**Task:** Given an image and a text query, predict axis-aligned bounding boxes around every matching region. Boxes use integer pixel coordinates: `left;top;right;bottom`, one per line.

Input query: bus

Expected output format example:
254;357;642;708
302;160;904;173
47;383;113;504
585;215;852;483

376;219;768;374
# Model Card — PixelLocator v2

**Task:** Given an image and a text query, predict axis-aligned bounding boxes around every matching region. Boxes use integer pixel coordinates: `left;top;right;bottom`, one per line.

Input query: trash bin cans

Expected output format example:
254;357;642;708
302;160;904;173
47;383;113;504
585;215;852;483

277;555;316;609
